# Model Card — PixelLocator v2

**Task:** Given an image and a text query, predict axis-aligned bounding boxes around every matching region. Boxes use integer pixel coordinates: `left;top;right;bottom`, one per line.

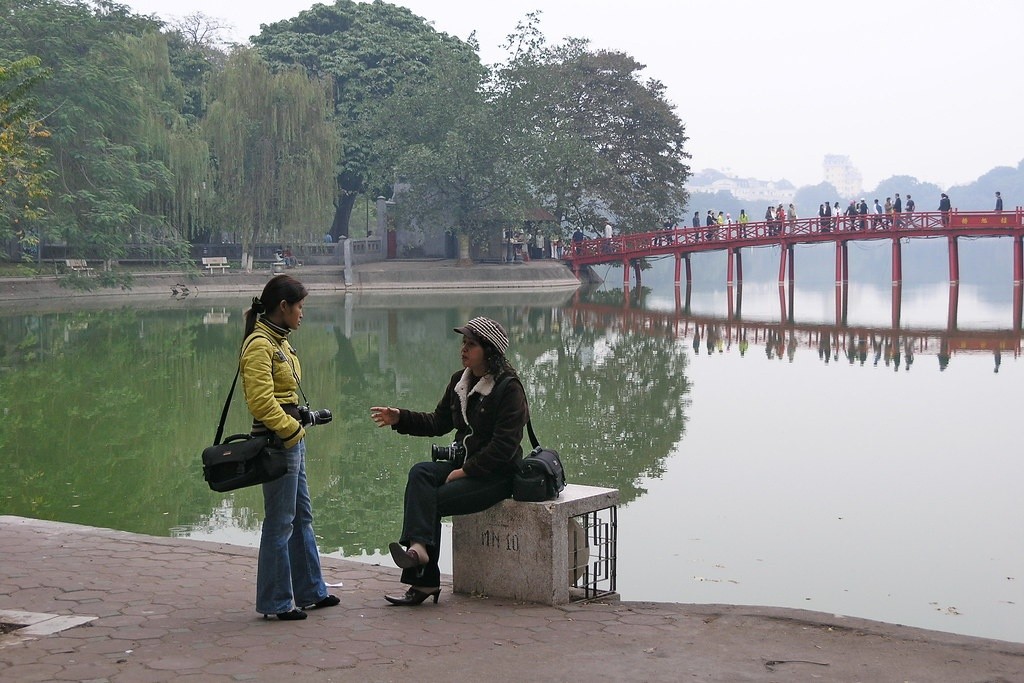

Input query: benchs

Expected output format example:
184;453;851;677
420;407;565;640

272;262;301;276
66;260;94;278
202;257;231;274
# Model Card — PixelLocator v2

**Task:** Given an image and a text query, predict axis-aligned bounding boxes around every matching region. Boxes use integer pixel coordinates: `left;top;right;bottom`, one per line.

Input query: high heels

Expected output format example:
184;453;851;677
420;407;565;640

302;595;340;610
384;586;442;605
389;542;428;577
264;608;307;620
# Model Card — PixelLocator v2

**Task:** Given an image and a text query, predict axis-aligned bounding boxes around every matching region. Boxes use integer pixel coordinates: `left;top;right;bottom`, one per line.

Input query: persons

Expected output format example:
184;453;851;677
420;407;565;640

766;203;797;237
994;192;1003;211
369;316;529;607
513;225;565;260
239;275;340;623
691;209;748;243
572;226;584;254
818;194;918;233
938;192;951;227
602;220;616;253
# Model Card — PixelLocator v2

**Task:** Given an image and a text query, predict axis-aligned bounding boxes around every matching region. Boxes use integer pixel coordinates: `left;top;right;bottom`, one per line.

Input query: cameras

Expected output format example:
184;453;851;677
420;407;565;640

430;441;466;465
297;403;333;427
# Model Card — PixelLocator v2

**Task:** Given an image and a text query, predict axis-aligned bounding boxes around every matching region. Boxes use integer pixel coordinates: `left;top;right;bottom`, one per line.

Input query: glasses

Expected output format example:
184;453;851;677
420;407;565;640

462;340;477;348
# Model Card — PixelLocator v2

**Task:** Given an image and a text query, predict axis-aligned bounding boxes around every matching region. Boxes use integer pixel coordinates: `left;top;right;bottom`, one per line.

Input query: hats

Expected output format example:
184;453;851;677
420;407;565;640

453;317;509;355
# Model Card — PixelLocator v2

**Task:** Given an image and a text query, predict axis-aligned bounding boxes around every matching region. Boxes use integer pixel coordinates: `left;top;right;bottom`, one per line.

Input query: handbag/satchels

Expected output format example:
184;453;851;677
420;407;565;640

512;445;567;502
202;434;287;492
